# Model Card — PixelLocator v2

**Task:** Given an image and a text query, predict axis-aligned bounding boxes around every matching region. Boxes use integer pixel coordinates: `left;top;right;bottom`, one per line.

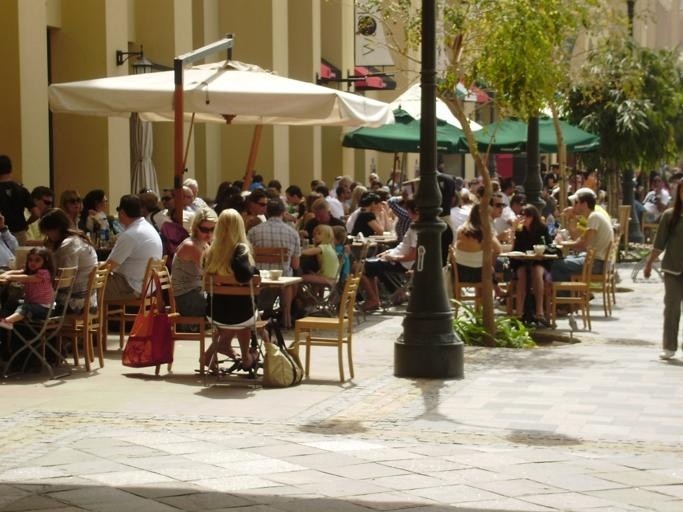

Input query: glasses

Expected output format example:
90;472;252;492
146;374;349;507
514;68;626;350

495;202;506;208
199;226;215;233
256;201;268;208
162;195;172;202
41;200;56;206
116;206;120;211
69;198;81;204
520;213;531;217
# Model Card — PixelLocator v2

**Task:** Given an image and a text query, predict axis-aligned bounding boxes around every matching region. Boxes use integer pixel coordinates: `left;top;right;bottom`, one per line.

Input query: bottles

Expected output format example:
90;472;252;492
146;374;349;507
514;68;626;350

99;217;110;250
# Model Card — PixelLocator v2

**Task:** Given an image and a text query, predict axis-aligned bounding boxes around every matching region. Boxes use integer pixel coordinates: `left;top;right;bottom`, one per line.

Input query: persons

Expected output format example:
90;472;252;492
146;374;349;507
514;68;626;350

634;175;671;226
438;160;613;328
644;180;683;360
0;157;417;370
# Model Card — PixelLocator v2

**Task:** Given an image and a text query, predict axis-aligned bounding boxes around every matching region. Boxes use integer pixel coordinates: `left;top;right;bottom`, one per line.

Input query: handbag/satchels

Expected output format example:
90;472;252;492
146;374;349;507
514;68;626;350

262;340;304;388
121;270;173;366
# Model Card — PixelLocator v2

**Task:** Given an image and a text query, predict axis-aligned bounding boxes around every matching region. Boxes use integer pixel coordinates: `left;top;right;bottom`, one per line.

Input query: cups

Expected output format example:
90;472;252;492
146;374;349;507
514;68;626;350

302;239;309;250
260;270;271;281
533;245;545;256
270;269;282;281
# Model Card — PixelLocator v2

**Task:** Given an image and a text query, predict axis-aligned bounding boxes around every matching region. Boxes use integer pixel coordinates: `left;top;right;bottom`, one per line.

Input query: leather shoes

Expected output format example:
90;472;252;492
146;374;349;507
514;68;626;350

521;293;594;328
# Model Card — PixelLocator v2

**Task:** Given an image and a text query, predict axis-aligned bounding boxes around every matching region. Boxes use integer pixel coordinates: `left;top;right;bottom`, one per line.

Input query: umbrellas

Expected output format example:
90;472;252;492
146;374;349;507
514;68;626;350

415;111;473;162
533;114;599;155
469;118;572;172
46;32;394;265
343;103;451;177
378;81;481;132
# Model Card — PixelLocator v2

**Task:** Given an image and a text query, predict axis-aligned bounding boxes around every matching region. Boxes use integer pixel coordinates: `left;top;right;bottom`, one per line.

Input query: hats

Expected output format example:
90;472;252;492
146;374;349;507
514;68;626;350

567;187;597;201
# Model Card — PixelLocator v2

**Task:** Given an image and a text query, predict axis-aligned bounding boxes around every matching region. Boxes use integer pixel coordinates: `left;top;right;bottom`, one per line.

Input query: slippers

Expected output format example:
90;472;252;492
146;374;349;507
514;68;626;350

199;352;219;371
361;301;379;312
243;348;260;371
218;347;240;360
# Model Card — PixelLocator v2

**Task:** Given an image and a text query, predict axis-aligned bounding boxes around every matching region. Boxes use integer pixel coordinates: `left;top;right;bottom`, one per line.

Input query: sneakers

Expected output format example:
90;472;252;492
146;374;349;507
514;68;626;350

393;295;408;305
660;349;676;360
303;305;319;314
1;318;14;329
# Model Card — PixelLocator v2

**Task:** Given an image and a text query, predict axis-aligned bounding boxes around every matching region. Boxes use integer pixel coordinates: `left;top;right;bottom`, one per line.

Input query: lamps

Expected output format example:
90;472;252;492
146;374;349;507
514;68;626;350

115;43;152;75
